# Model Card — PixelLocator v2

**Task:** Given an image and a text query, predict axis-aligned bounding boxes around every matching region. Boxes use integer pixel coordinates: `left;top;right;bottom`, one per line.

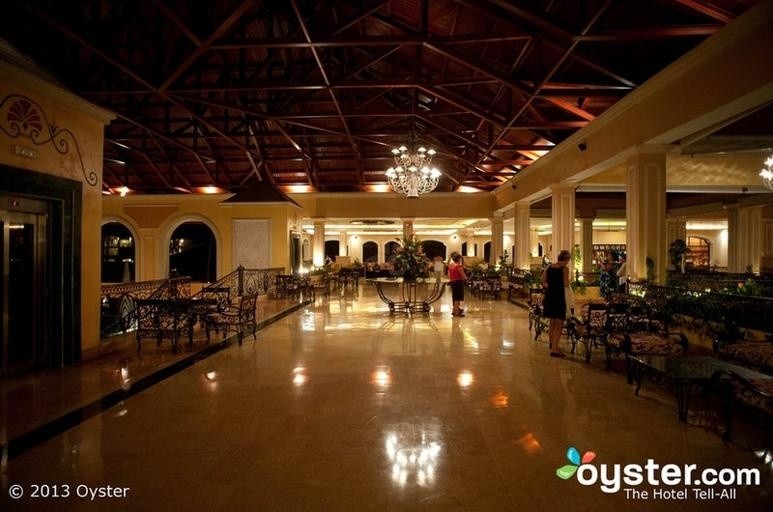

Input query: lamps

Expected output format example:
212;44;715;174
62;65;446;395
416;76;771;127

386;414;447;489
385;131;442;199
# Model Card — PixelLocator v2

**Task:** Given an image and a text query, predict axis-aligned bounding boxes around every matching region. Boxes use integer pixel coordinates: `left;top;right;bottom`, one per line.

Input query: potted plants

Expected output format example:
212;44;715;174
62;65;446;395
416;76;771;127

389;232;432;283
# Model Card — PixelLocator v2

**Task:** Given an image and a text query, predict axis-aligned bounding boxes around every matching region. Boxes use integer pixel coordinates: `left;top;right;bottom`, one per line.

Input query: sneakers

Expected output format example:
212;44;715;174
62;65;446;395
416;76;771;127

550;352;566;357
452;308;464;317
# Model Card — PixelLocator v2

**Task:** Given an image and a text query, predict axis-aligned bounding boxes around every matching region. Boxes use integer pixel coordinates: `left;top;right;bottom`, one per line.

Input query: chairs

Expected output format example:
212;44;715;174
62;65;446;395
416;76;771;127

135;285;259;350
463;263;543;300
272;267;360;302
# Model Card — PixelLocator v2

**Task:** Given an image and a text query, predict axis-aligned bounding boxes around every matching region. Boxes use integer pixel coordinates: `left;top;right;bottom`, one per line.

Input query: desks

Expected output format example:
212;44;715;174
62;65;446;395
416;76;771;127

366;276;452;316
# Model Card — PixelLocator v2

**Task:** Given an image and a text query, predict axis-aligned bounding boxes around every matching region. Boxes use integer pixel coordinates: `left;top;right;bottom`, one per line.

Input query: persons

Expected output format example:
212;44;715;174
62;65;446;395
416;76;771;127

447;251;471;277
596;250;620;304
430;252;446;283
494;255;504;272
614;253;626;294
479;260;488;269
324;254;332;265
447;253;467;318
541;249;572;358
